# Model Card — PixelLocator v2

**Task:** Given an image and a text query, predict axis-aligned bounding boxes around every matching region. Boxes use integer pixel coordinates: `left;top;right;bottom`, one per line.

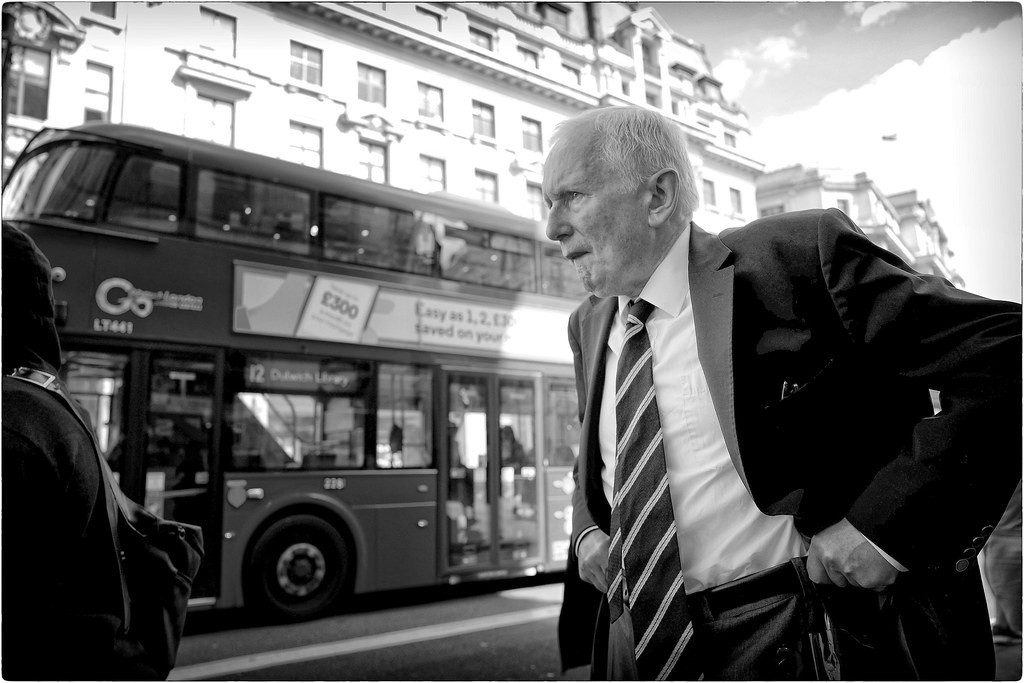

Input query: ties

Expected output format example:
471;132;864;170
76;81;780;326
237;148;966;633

607;298;704;681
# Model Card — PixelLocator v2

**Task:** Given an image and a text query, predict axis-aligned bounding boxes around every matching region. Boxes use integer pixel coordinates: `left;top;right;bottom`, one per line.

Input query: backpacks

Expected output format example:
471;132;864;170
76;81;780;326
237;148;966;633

2;367;205;681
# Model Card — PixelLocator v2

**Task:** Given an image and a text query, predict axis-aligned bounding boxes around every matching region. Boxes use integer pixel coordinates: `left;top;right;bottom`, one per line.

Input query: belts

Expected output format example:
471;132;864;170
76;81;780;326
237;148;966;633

685;559;800;622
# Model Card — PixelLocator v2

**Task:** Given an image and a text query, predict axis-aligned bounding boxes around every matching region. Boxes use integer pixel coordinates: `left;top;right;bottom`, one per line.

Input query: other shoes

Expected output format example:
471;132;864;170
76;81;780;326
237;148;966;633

992;625;1022;645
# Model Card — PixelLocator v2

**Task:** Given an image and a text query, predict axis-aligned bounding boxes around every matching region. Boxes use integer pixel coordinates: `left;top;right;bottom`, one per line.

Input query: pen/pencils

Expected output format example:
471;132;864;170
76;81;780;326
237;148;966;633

791;384;799;394
781;381;789;400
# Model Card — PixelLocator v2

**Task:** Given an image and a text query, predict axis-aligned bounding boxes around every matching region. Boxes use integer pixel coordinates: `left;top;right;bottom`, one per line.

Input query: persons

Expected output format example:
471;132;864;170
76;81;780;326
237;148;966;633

539;105;1022;682
2;222;137;681
443;421;527;549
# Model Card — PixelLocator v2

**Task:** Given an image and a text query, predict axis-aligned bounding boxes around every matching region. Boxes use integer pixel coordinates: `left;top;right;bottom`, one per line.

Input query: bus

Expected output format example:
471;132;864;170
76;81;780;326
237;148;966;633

2;120;590;629
